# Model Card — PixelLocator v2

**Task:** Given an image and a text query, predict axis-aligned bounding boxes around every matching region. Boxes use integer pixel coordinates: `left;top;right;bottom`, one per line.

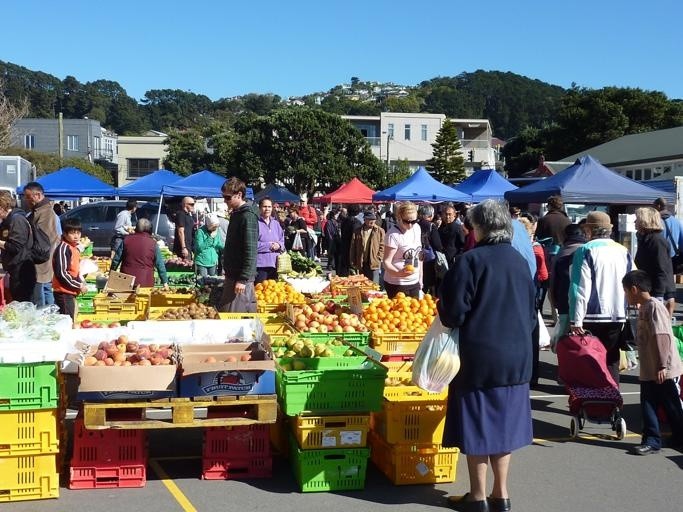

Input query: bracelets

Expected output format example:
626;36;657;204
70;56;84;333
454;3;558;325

181;246;187;250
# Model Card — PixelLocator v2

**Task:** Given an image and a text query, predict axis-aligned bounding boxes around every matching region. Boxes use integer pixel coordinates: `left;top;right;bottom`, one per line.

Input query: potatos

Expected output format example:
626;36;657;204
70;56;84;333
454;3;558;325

157;300;216;320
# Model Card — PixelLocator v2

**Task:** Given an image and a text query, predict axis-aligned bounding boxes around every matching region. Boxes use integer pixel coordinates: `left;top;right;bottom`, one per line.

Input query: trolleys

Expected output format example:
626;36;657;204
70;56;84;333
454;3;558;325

556;317;629;444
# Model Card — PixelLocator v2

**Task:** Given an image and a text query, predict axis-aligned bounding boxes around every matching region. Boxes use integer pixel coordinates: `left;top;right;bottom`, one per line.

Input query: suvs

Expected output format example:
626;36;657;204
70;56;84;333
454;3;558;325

56;199;175;253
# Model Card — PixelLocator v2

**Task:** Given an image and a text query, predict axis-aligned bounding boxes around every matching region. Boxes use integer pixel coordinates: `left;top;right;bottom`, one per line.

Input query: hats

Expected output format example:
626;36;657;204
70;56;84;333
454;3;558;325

301;197;306;201
215;208;226;218
205;213;220;230
580;211;613;228
363;210;376;220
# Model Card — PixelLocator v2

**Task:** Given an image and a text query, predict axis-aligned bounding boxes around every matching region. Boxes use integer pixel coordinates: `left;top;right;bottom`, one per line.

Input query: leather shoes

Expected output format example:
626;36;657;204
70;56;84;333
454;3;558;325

446;493;511;512
631;443;660;455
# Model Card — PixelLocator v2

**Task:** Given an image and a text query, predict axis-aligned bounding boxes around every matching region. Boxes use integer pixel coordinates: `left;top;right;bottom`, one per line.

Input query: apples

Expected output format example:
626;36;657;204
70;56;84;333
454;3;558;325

295;300;371;333
81;317;120;328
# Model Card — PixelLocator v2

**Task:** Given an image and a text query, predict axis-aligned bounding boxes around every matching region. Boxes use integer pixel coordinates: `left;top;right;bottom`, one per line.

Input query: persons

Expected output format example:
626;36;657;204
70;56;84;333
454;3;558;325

108;198;137;261
0;189;37;301
194;177;683;388
621;270;683;457
435;196;538;512
110;217;169;289
52;218;89;319
173;196;195;262
22;181;62;307
52;200;68;216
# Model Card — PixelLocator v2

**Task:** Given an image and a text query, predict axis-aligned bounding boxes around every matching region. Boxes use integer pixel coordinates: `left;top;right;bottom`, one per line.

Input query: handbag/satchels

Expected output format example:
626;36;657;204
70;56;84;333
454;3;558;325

276;251;292;274
620;303;638;351
422;234;436;263
671;249;683;274
332;231;346;256
434;251;449;279
538;309;551;348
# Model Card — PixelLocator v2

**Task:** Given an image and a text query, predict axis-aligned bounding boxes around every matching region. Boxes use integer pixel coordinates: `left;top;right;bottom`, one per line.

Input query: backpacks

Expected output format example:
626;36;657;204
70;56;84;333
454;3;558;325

32;223;51;264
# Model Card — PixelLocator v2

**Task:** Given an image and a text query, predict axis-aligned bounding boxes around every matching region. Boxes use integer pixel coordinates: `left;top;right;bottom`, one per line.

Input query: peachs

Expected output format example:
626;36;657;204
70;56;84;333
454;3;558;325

204;354;255;363
86;334;171;365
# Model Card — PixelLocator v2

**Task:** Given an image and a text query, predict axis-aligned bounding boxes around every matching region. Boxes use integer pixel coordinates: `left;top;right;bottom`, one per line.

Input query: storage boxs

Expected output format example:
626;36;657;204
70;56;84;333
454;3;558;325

67;341;282;401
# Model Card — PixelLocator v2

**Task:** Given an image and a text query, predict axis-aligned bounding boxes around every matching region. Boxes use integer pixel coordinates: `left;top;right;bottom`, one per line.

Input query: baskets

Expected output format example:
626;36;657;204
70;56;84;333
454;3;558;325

0;361;146;503
75;255;220;325
201;277;460;494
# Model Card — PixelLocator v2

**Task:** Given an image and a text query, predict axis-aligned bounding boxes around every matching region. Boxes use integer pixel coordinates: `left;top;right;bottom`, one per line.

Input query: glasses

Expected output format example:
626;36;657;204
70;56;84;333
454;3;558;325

223;193;235;200
401;216;420;224
186;203;194;207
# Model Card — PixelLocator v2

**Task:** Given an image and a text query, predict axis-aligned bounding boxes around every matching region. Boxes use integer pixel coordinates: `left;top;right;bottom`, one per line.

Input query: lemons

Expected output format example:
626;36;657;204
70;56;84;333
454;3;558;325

365;293;437;333
253;279;303;306
